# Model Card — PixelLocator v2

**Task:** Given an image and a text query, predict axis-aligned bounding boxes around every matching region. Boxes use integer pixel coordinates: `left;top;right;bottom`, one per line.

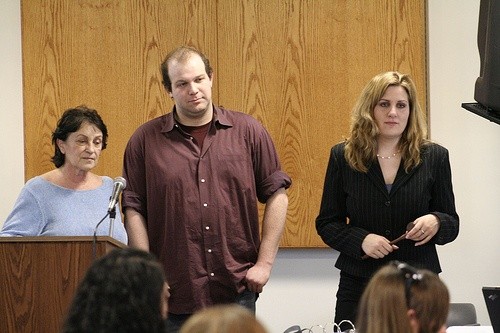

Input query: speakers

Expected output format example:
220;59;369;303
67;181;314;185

473;0;500;112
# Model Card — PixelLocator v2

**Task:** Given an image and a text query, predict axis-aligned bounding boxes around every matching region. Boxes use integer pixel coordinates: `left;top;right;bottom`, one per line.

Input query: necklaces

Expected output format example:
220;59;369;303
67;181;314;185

376;148;402;158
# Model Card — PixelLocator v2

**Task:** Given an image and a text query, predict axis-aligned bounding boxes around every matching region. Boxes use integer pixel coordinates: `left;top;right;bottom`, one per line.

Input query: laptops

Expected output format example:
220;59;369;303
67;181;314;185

482;287;500;333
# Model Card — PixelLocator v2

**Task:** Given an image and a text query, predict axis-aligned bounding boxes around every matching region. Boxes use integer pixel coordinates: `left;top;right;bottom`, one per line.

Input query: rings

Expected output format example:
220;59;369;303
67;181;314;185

420;228;425;233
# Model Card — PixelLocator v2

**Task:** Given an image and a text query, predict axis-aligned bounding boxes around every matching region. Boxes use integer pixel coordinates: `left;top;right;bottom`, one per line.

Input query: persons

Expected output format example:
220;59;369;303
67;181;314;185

0;105;129;247
121;45;292;327
316;71;460;333
63;245;270;333
355;259;450;333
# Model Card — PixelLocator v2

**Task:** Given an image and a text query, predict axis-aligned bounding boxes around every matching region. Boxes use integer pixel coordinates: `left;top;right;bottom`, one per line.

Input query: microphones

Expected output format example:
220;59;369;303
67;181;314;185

108;177;127;212
